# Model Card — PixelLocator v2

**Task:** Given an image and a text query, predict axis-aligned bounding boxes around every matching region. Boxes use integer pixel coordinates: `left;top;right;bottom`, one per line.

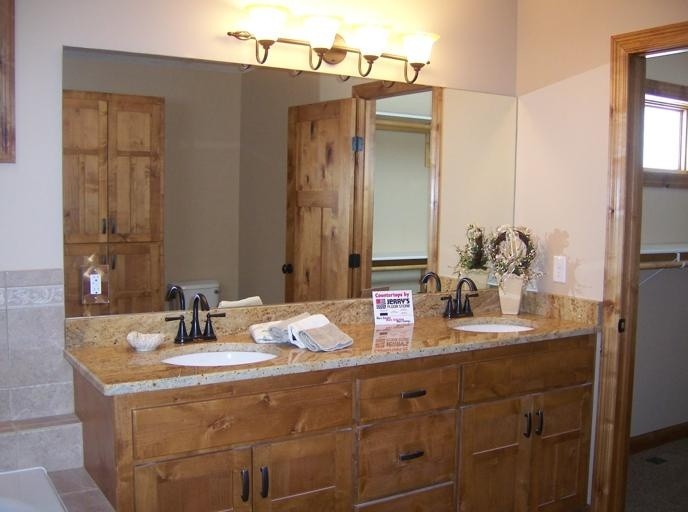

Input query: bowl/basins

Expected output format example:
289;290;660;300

127;331;164;352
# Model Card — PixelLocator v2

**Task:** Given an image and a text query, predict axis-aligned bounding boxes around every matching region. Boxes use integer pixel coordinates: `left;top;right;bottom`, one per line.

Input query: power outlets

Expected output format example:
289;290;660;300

552;256;567;284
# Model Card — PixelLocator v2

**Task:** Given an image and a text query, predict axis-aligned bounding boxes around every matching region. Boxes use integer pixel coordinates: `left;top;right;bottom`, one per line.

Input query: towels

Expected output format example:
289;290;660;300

250;312;355;354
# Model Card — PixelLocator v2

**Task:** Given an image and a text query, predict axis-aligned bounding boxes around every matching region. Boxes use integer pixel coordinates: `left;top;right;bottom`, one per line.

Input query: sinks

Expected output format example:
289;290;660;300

159;341;280;367
446;316;539;333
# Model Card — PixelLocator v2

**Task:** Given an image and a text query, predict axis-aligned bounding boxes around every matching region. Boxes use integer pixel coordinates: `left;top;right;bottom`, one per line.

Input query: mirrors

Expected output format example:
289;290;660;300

61;46;518;324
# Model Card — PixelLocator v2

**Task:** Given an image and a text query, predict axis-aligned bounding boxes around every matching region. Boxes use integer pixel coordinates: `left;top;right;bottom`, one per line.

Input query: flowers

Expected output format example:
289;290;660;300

452;224;494;275
485;226;547;290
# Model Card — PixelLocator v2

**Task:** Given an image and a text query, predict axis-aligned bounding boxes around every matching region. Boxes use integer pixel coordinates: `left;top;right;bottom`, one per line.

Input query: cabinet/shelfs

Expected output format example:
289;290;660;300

358;354;462;510
62;88;166;317
463;334;594;512
73;367;355;511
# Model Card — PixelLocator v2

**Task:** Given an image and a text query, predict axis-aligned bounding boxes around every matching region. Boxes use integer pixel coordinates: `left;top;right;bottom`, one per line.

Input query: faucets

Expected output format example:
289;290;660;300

188;293;211;337
453;277;478;317
420;271;441;291
165;285;184;310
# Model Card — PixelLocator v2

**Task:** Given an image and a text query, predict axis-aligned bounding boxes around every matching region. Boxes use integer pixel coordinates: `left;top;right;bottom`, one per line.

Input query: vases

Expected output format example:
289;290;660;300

496;275;524;314
465;269;486;290
